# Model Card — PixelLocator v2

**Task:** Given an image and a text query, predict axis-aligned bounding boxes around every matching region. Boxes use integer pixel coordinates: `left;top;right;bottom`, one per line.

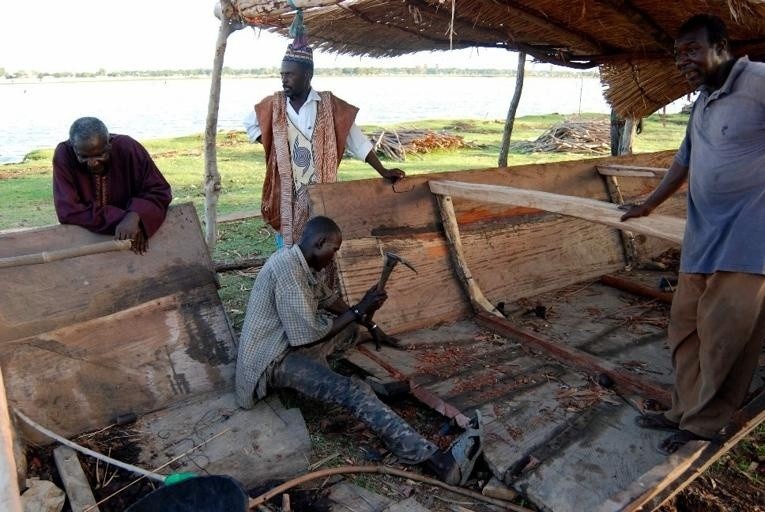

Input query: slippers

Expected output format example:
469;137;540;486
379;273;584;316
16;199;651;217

634;413;679;431
655;432;711;455
441;408;485;487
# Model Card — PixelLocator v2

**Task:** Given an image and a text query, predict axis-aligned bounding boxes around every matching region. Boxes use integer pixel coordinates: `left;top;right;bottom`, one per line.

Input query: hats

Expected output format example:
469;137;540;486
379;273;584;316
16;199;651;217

282;43;314;68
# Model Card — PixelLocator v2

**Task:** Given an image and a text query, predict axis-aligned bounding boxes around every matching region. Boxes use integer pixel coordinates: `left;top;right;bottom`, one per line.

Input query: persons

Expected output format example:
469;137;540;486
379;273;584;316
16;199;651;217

53;117;172;257
234;216;484;489
617;14;764;456
246;38;405;245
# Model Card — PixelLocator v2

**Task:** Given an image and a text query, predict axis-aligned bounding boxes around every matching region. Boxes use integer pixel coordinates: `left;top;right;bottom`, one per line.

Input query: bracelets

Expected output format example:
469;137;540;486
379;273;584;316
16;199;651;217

348;306;362;320
369;324;378;331
